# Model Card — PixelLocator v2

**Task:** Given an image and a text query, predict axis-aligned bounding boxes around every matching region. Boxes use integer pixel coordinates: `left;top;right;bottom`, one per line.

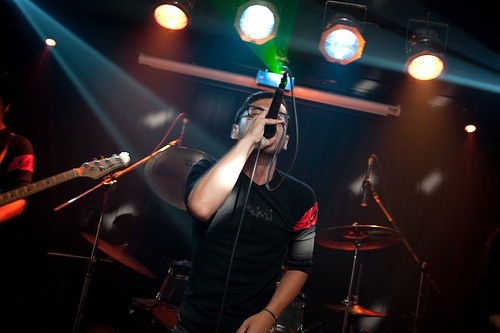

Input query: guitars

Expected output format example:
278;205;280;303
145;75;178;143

0;152;131;206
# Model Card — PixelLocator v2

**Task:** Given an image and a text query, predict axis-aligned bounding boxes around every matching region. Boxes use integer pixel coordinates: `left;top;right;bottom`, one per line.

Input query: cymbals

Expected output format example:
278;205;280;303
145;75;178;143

81;230;156;279
143;146;218;210
313;301;382;320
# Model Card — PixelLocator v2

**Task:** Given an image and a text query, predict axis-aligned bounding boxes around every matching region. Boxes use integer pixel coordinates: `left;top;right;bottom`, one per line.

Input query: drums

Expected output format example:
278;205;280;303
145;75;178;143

316;222;403;251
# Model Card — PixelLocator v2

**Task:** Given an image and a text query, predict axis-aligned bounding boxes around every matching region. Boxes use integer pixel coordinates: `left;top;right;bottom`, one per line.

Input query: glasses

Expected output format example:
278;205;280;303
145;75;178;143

236;105;290;121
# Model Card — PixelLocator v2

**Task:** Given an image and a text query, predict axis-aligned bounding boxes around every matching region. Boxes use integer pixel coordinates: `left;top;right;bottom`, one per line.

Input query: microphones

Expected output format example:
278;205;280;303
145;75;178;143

178;116;189;147
361;155;374;207
264;70;287;140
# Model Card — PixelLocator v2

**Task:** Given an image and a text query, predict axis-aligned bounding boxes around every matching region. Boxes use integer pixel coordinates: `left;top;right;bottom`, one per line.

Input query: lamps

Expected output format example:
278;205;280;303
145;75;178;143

318;0;367;64
151;0;194;31
403;17;450;81
233;0;281;45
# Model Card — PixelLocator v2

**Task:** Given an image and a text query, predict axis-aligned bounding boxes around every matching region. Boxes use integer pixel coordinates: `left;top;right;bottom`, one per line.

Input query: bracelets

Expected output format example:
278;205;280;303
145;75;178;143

263;307;278;322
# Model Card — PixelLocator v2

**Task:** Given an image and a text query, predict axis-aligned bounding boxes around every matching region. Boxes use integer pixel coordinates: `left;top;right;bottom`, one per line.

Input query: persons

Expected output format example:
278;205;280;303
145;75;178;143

176;91;319;333
0;84;34;224
